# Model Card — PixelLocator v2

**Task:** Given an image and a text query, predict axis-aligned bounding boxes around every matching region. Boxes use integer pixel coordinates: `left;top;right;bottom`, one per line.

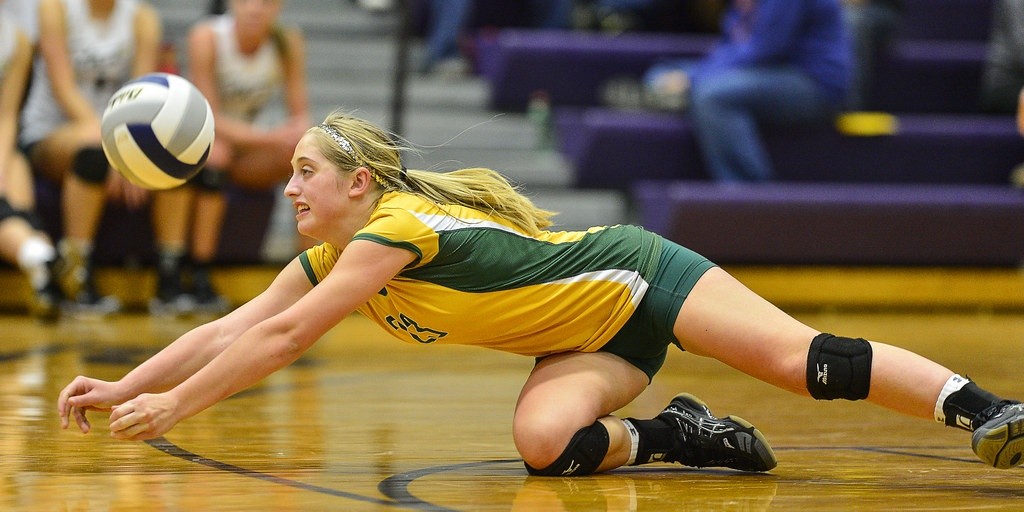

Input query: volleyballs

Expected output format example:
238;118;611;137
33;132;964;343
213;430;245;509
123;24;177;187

99;65;218;192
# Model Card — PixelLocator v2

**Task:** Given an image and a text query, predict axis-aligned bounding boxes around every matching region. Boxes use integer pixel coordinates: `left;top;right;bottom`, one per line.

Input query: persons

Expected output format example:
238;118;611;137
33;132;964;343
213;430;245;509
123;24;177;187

687;1;855;185
0;0;315;324
55;108;1024;512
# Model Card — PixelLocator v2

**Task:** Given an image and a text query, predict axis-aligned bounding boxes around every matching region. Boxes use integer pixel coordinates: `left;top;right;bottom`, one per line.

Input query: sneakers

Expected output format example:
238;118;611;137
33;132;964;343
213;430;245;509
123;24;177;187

971;399;1024;469
657;392;778;471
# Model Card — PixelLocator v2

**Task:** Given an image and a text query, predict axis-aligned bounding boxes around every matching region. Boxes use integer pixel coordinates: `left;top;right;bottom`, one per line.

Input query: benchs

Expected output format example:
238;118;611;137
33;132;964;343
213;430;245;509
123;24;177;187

448;0;1022;272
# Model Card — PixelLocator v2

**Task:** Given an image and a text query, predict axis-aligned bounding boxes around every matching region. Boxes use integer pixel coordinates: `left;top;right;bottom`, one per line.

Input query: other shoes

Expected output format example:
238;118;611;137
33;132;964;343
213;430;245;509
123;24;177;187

194;286;217;303
43;283;65;302
158;275;179;301
86;286;100;304
